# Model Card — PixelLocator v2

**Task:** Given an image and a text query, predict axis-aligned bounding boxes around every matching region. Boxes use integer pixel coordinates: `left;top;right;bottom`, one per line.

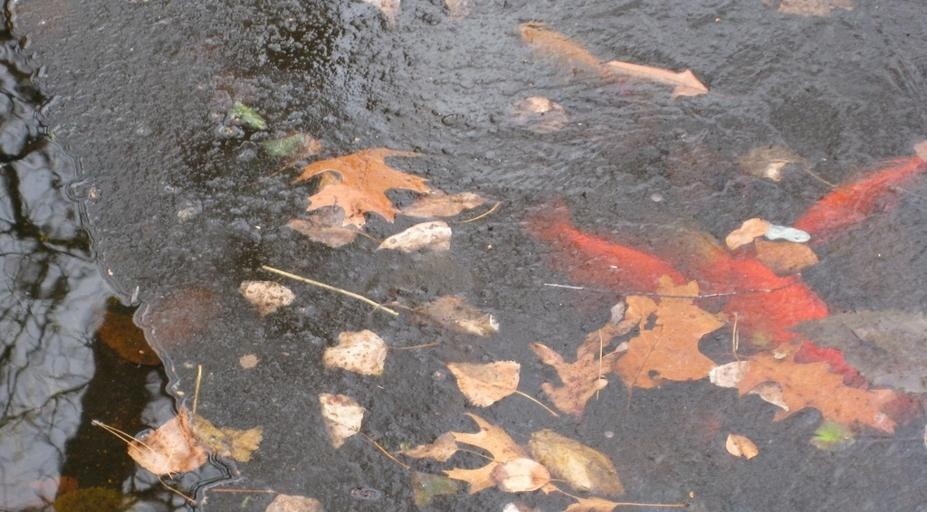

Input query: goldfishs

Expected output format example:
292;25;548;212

524;196;915;422
